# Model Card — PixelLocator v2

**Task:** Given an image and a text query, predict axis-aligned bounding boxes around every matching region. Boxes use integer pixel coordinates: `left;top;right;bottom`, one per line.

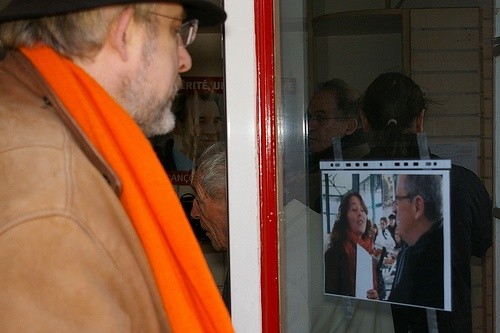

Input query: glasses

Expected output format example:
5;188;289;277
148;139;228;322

308;111;355;127
394;193;418;206
135;8;199;49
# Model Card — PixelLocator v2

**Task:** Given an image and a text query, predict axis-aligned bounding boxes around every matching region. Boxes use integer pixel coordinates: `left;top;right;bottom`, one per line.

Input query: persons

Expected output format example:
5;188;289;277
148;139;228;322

0;0;236;333
325;173;446;309
284;78;373;210
358;73;494;333
190;141;395;333
161;89;223;171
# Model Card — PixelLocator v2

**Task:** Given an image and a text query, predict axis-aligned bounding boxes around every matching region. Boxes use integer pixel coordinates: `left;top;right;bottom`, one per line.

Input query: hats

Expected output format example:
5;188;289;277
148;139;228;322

0;0;227;28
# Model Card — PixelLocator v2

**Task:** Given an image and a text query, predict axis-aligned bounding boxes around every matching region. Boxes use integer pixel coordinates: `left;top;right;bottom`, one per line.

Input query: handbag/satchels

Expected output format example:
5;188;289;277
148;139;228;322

180;193;207;239
